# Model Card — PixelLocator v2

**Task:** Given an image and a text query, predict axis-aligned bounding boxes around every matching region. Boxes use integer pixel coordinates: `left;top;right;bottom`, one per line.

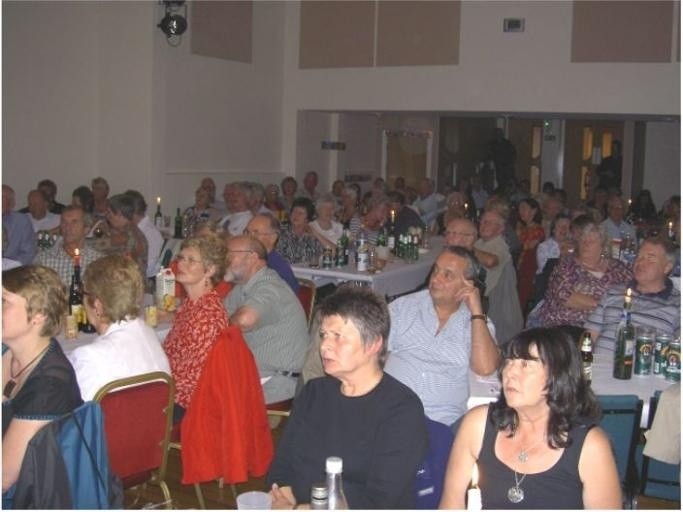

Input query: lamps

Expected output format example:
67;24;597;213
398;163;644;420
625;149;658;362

157;0;188;47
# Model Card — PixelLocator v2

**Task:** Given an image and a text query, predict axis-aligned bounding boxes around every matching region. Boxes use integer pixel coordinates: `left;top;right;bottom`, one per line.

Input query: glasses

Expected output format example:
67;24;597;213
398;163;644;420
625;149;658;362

175;229;272;266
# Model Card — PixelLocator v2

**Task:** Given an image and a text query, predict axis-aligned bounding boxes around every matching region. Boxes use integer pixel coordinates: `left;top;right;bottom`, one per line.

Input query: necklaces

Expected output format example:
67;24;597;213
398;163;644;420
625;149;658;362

508;434;548;463
507;461;529;505
4;342;49;399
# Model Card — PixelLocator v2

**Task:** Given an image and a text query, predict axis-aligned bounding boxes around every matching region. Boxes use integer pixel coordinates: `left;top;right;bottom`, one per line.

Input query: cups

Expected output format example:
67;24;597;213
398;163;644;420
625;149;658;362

610;239;622;259
64;315;79;341
236;491;273;510
309;249;320;269
144;305;157;328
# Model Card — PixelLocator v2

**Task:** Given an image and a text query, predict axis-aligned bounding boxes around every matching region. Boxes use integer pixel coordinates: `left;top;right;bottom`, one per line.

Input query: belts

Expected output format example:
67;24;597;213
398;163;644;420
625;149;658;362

274;368;302;378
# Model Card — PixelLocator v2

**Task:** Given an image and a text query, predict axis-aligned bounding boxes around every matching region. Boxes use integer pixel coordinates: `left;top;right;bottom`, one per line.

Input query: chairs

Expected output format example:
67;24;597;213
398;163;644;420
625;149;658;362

265;399;293;418
92;372;178;510
296;277;316;331
596;395;644;509
636;391;682;510
8;401;103;508
170;323;243;510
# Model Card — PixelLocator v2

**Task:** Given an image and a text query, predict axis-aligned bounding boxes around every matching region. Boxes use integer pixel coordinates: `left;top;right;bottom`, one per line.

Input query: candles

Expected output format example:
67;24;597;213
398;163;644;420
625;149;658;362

157;196;161;204
625;289;631;302
391;210;394;224
468;463;481;511
74;249;81;265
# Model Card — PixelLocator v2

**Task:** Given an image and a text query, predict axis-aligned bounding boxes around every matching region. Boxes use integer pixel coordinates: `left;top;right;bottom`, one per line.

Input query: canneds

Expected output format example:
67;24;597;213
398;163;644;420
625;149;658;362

666;339;681;381
634;337;653;375
336;246;345;266
322;246;332;269
654;336;670;378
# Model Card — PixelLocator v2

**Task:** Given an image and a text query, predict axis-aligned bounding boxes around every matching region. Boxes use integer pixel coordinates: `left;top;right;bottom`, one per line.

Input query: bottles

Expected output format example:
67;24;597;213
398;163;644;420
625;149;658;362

174;208;183;238
309;455;349;510
154;205;162;228
620;217;645;261
69;266;96;333
324;209;430;271
613;287;635;380
580;332;593;383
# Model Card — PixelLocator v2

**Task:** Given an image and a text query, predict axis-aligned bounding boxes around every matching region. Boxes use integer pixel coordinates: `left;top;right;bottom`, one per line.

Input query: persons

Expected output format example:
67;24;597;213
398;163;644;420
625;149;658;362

581;234;681;355
3;264;86;509
263;280;427;510
2;170;681;327
569;138;623;200
66;254;171;476
436;326;623;509
224;235;310;406
160;229;230;443
379;246;503;425
